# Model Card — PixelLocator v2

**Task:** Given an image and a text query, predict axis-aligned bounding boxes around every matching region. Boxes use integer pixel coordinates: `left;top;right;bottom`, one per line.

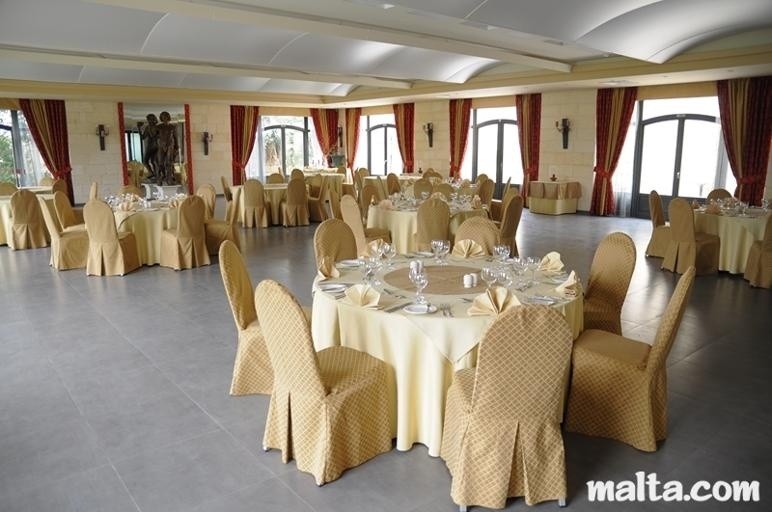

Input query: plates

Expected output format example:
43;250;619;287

404;304;437;314
547;276;578;285
411;251;434;258
521;296;555;305
319;283;346;292
341;260;361;266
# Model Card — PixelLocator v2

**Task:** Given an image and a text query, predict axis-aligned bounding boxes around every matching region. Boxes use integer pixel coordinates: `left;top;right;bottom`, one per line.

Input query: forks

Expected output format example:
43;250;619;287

445;304;455;317
439;303;448;317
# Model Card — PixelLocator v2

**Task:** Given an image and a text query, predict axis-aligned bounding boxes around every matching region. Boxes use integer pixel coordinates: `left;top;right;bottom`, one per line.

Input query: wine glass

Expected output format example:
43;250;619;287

389;190;417;209
421;192;430;200
415;272;428;304
493;247;505;267
497;269;514;289
459;195;466;208
372;245;380;264
438;240;449;266
498;244;511;265
513;256;528;289
450;193;458;209
409;261;423;300
383;243;396;265
527;256;541;284
432;239;443;260
481;268;497;287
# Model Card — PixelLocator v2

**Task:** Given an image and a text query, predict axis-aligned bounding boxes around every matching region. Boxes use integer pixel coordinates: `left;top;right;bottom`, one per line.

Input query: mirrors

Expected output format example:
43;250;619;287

117;102;193;195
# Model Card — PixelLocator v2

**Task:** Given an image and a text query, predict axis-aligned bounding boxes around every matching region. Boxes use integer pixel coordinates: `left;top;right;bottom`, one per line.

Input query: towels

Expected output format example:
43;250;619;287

314;255;340;283
691;199;700;207
468;286;524;316
430;192;447;200
379;199;392;209
364;239;395;261
451;240;485;259
558;270;583;299
344;284;383;309
537;251;564;272
118;201;135;211
170;201;183;208
473;194;481;208
705;205;720;212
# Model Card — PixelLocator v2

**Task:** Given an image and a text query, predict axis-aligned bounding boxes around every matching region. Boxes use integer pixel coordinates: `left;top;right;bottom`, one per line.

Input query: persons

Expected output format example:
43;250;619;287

148;110;176;186
135;113;159;181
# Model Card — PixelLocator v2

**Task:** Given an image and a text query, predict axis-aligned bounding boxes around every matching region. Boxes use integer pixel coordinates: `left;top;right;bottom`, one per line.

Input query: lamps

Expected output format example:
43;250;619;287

201;132;214;155
338;126;343;148
423;123;434;147
96;124;110;151
556;119;571;148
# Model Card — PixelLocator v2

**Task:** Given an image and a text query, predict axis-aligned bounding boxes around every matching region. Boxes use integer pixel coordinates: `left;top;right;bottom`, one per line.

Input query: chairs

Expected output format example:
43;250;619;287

52;178;67;196
355;168;363;202
500;195;524;258
314;218;358;269
280;179;310;228
182;183;217;229
434;184;455;201
490;177;512;220
268;173;284;183
328;186;343;221
337;166;347;183
39;177;55;187
387;173;402;195
0;182;18;196
53;191;85;232
119;185;146;198
220;176;233;224
38;196;89;271
8;189;48;249
441;302;574;512
645;190;670;258
219;240;313;396
660;197;720;276
204;187;241;255
363;185;380;217
376;175;388;201
743;212;772;288
565;266;697;452
340;194;392;256
423;167;443;183
290;169;305;183
413;179;434;199
240;179;272;227
493;187;519;232
706;185;732;207
474;174;488;187
83;200;140;276
72;181;99;223
159;196;211;271
453;217;504;256
584;232;637;336
479;179;495;219
413;199;450;252
254;279;392;486
359;168;370;175
